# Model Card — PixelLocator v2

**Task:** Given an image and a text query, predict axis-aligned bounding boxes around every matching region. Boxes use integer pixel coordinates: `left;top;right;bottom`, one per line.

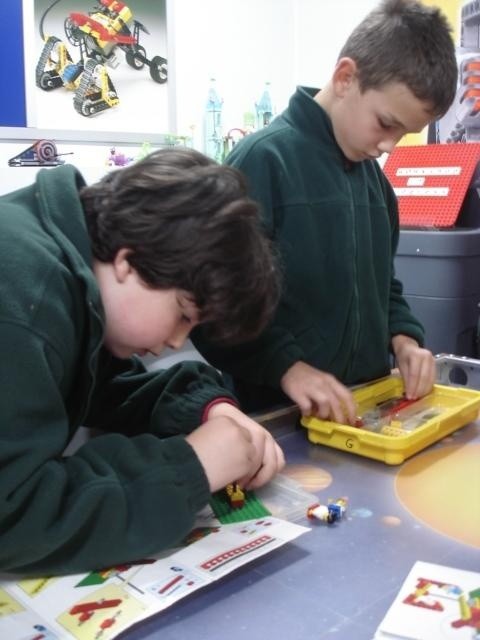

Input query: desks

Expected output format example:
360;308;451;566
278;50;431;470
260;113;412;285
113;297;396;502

0;352;480;640
393;229;480;352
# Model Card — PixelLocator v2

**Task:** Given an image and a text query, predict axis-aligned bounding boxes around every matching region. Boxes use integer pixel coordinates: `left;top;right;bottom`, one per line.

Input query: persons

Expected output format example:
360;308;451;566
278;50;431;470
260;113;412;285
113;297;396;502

188;0;460;427
2;142;284;580
